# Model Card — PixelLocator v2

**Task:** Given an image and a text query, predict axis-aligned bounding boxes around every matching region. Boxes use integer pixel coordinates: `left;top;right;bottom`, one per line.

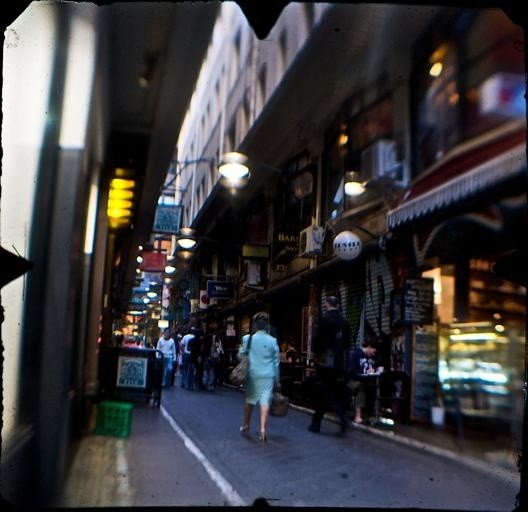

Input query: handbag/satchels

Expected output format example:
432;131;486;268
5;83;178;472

229;334;252;386
269;385;289;417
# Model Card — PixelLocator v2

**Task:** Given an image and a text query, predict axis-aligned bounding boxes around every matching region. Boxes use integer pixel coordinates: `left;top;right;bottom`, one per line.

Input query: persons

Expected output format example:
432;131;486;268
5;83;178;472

351;341;377;421
307;294;352;439
156;326;224;392
236;313;280;444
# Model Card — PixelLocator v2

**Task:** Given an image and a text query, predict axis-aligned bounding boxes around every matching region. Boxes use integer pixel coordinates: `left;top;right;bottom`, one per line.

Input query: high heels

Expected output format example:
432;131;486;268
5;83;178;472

239;424;249;435
258;432;266;441
